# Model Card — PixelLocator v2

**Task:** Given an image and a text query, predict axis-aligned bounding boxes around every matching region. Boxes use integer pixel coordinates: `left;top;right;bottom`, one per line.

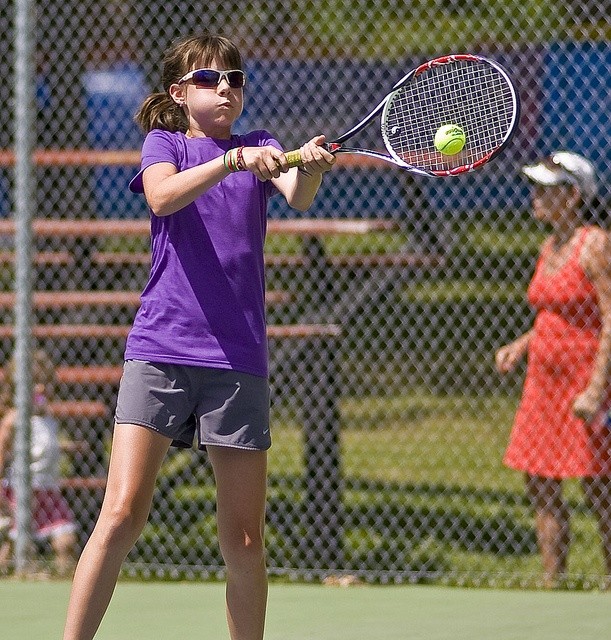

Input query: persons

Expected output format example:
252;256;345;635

496;150;611;590
61;35;337;640
0;347;78;577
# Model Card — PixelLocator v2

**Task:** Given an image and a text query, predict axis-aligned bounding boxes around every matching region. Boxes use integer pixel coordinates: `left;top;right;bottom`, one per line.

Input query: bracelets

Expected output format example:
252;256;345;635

223;145;249;176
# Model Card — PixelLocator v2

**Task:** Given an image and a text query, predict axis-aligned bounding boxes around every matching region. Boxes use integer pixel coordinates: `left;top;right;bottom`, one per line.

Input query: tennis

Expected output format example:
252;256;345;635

434;124;465;155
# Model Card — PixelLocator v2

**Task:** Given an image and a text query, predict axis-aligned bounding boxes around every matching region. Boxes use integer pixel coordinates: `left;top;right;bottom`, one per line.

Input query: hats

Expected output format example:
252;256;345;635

522;151;596;208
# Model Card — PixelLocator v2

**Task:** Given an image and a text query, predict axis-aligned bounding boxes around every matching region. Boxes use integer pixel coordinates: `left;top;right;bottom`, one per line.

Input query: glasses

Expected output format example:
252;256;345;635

178;69;245;87
